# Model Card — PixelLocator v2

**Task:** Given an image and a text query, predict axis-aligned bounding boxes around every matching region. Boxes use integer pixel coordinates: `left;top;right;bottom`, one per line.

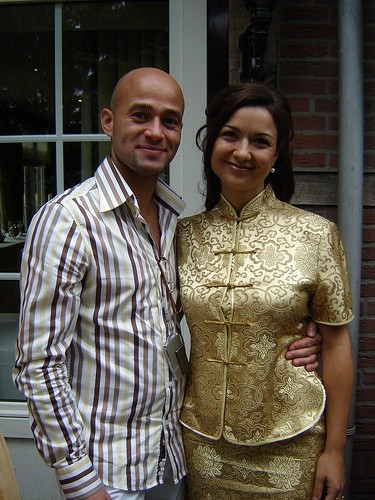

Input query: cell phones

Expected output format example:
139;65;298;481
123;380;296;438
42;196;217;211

163;333;189;383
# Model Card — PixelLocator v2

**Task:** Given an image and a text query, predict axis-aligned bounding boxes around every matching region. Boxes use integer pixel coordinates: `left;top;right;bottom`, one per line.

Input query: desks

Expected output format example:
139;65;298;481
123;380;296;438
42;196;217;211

0;233;28;314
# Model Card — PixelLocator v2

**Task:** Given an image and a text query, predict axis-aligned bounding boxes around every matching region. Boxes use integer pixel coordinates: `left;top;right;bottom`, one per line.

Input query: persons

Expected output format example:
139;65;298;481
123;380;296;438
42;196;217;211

10;66;323;500
174;82;355;500
